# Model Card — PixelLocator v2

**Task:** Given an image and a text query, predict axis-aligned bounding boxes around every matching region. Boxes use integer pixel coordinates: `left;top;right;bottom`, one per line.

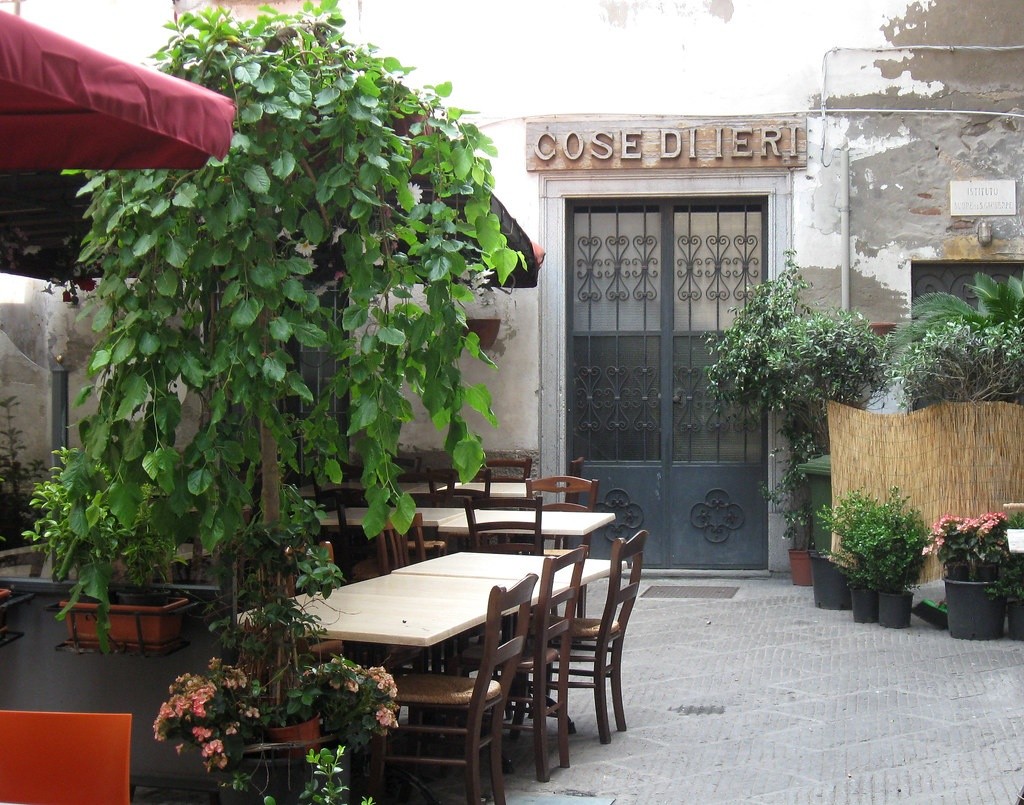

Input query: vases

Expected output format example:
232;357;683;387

973;561;999;581
943;576;1006;640
266;709;321;761
946;562;969;581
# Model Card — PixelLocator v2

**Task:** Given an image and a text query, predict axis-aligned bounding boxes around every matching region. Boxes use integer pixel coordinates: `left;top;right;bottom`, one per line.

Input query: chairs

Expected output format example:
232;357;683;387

112;443;648;805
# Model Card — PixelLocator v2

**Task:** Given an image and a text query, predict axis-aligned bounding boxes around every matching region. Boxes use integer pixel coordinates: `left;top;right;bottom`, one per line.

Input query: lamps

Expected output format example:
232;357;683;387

977;217;993;246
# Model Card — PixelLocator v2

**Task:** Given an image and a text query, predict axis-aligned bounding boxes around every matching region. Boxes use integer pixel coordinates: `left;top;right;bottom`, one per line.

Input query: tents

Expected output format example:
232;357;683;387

0;9;236;169
0;163;540;289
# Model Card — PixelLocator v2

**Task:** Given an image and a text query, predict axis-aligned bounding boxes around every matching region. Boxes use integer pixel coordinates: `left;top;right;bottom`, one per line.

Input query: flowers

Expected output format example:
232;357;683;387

152;653;270;774
921;512;1012;561
284;654;401;756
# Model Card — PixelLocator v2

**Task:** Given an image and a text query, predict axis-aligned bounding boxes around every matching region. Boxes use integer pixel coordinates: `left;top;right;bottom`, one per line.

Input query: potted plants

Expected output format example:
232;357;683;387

843;489;929;630
761;466;817;587
983;553;1024;641
1005;511;1024;553
817;491;888;623
21;0;523;805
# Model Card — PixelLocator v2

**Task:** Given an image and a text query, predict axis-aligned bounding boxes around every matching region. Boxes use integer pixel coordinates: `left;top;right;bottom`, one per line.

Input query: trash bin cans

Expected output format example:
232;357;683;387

797;454;832;552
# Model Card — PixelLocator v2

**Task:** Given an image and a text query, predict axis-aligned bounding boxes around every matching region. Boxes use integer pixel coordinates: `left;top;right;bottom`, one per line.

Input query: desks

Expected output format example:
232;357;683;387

291;480;425;501
438;510;616;556
391;551;628;741
319;508;481;583
222;574;540;805
406;480;539;498
